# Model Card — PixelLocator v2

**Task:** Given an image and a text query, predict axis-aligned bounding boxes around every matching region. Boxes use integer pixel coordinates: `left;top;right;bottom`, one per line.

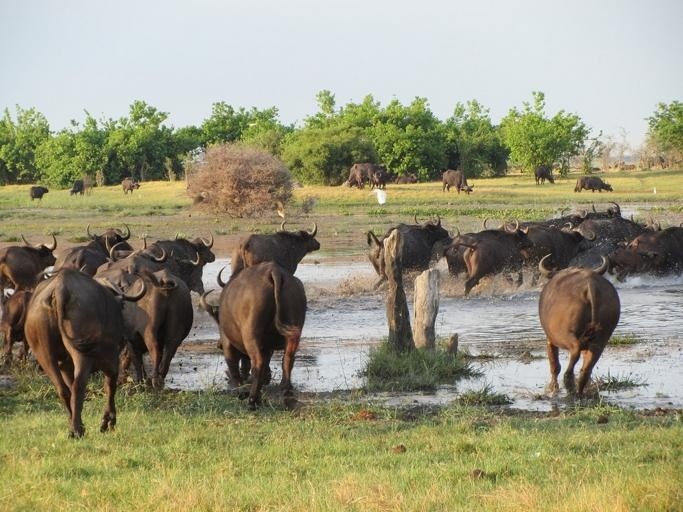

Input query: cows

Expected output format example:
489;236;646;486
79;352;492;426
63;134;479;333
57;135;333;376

120;176;141;195
69;178;84;196
440;168;475;196
30;185;50;201
1;220;321;441
573;175;615;194
346;162;418;189
362;200;683;396
534;165;557;186
